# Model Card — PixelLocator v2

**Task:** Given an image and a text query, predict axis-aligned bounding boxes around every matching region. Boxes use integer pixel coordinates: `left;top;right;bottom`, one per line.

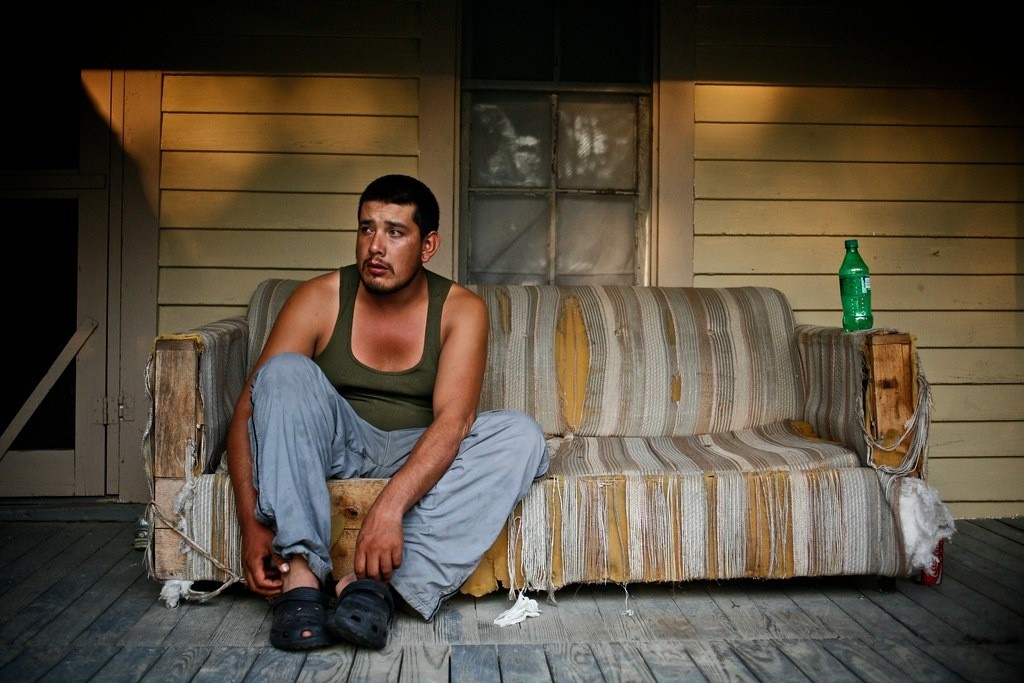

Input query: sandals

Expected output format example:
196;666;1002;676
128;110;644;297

271;578;394;652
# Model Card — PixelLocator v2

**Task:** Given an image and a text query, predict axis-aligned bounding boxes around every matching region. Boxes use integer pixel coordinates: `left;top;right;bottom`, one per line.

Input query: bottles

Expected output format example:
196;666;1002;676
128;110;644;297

839;240;875;331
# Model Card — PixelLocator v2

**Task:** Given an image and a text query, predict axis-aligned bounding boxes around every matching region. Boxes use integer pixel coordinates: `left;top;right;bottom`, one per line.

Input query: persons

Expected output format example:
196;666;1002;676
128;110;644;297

228;174;549;653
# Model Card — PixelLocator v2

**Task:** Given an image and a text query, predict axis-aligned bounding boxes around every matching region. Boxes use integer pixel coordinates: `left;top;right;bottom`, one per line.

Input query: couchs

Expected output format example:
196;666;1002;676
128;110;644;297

140;277;956;630
